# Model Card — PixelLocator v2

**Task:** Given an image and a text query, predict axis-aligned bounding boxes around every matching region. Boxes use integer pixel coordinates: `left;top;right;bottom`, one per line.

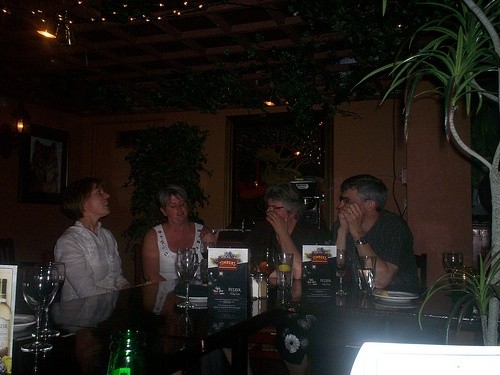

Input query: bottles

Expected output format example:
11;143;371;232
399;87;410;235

248;272;259;299
257;272;267;300
106;327;146;375
0;279;12;358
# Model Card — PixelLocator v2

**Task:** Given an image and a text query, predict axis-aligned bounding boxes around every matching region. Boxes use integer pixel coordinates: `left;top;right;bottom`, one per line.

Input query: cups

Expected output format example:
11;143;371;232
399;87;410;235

200;242;215;260
356;255;377;296
275;252;294;292
246;248;257;272
442;250;463;273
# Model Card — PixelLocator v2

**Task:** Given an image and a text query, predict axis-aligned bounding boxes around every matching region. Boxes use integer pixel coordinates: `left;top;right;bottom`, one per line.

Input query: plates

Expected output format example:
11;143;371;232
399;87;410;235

176;294;207;300
371;290;419;303
14;313;37;332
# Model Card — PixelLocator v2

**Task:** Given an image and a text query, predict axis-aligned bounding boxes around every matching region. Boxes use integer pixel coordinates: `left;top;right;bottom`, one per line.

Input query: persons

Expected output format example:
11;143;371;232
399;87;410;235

54;177;131;302
330;174;420;294
143;279;193;353
51;291;119;375
244;183;317;279
142;186;215;283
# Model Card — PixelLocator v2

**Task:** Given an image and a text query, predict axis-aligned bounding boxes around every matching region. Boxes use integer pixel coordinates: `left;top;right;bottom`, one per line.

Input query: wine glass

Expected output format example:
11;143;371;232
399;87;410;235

20;265;60;353
31;260;66;338
334;249;352;295
258;248;278;300
176;247;199;309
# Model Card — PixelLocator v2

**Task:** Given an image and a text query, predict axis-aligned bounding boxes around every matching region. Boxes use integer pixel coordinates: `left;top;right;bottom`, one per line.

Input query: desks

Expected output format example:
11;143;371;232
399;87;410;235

0;279;486;375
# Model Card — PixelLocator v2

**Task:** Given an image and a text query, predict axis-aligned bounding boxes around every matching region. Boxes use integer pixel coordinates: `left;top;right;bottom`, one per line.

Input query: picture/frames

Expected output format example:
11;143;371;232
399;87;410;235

16;123;70;205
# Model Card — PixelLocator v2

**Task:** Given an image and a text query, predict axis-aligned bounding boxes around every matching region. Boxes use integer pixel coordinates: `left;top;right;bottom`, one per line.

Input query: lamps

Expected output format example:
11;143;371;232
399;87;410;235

263;95;280;108
0;107;31;160
36;7;75;46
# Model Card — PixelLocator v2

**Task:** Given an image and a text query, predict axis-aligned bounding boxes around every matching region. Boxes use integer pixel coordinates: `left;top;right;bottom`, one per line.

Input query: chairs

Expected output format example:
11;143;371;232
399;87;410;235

415;253;427;291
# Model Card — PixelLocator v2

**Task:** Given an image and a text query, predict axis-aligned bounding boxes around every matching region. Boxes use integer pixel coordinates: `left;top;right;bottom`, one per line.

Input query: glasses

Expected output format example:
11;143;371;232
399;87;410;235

339;196;365;205
272;206;284;210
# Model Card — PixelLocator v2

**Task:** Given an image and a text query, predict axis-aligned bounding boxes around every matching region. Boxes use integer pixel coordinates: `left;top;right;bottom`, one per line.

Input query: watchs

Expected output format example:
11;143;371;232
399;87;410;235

355;237;368;245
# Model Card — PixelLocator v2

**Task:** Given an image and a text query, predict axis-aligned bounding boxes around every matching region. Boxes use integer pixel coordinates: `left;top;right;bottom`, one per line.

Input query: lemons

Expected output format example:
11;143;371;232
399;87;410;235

278;264;291;272
380;290;388;296
